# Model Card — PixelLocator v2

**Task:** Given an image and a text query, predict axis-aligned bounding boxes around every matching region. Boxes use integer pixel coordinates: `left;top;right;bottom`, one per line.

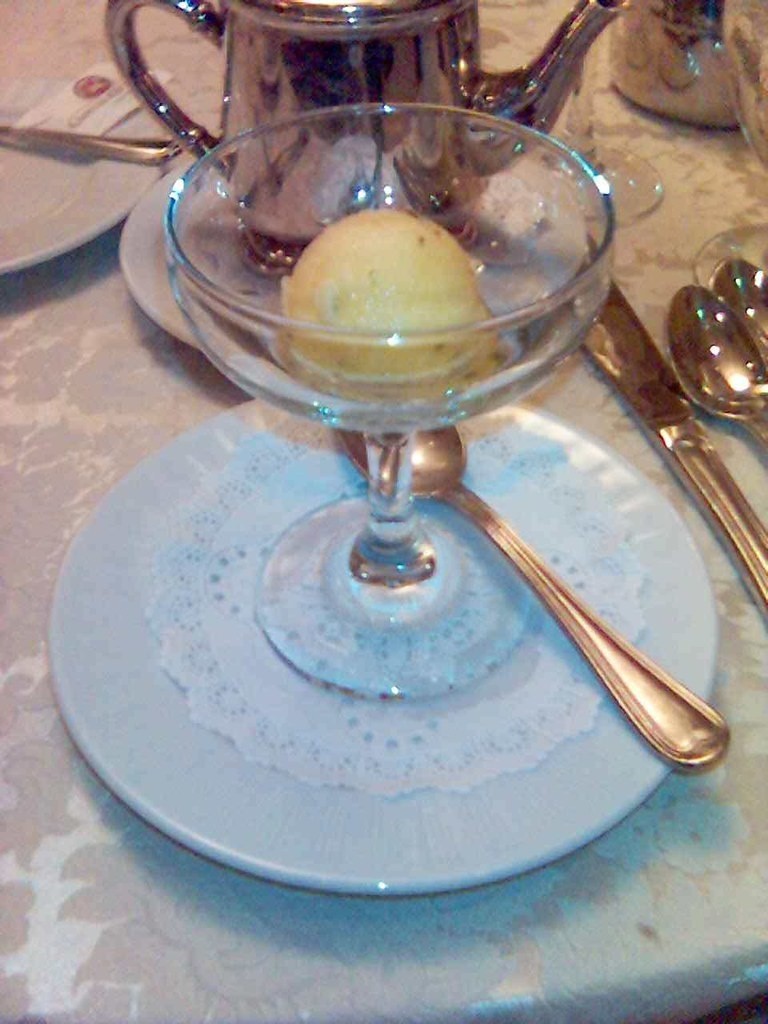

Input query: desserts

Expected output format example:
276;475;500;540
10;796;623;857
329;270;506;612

278;204;502;400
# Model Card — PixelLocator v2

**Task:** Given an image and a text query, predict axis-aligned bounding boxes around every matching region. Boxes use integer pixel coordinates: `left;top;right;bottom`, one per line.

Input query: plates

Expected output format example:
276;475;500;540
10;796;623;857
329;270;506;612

48;397;718;897
0;73;166;276
118;157;584;350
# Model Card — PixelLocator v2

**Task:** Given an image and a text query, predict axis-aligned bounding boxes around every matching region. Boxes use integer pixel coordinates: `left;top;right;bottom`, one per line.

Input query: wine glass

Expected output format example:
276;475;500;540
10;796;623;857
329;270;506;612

521;48;665;229
693;0;768;285
161;102;613;701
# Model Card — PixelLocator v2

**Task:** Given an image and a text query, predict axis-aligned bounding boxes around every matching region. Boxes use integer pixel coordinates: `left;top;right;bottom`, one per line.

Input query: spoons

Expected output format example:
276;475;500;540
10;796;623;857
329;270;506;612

666;283;768;452
708;257;768;377
334;424;732;768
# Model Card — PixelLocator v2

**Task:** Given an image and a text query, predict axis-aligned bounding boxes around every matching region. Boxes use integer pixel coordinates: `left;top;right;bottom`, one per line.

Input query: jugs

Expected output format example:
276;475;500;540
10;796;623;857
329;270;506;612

105;0;623;278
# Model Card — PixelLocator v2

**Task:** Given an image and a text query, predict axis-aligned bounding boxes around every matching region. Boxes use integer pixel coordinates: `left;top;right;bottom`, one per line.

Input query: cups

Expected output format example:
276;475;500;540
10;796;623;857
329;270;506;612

609;0;739;128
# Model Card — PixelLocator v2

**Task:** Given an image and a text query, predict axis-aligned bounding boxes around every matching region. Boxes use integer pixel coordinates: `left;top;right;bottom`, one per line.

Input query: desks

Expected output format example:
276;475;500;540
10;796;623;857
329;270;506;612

0;0;768;1022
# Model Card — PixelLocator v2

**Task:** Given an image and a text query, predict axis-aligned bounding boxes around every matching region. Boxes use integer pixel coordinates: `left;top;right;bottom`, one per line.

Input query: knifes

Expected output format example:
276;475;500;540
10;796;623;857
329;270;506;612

566;235;768;612
